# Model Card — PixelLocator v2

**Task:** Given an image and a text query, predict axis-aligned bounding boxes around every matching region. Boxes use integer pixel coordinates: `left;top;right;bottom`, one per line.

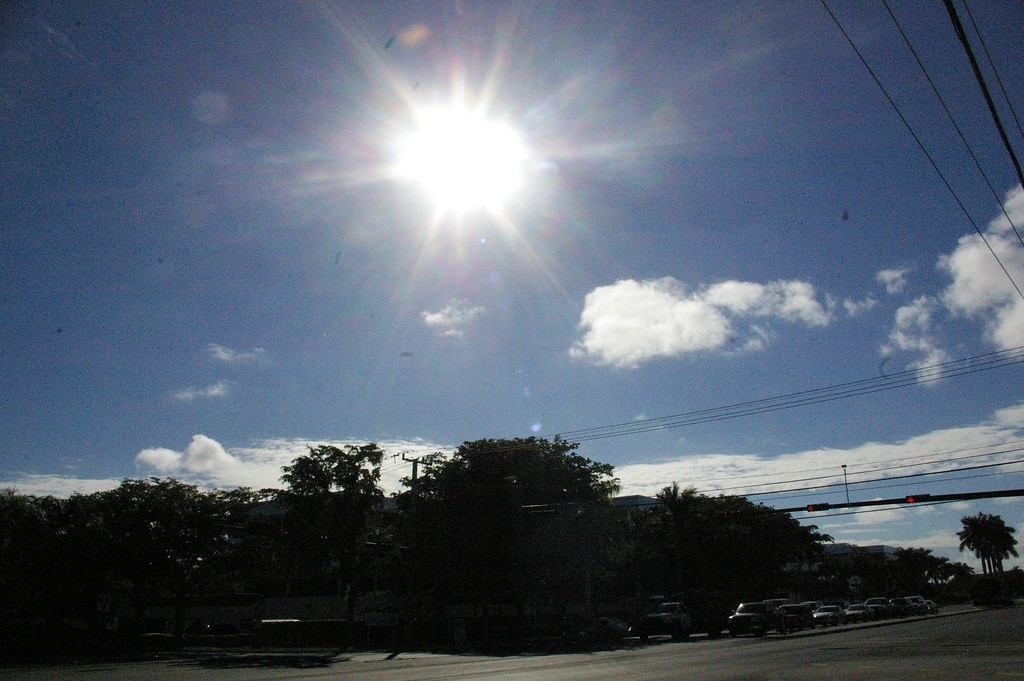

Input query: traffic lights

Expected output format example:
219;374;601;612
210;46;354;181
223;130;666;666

806;502;830;512
906;493;929;504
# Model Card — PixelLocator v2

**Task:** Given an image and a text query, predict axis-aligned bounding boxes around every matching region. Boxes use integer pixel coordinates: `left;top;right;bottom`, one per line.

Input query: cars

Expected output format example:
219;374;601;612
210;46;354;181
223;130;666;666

575;618;627;637
727;594;940;637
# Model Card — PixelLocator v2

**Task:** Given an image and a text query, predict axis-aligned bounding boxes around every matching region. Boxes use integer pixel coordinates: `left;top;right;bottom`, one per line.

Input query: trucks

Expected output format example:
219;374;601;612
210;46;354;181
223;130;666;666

636;602;727;640
